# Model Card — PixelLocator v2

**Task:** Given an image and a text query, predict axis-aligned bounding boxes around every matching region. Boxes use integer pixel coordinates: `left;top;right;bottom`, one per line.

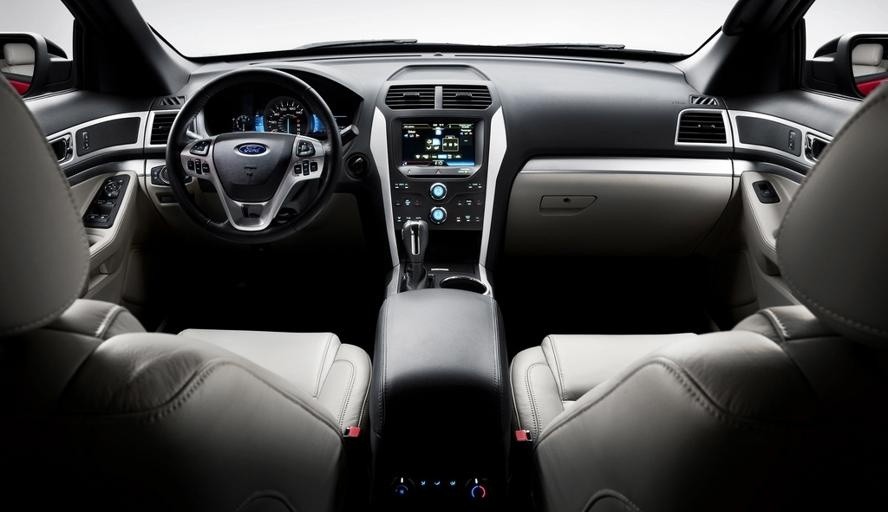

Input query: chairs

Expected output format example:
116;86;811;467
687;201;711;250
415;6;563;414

0;73;375;511
510;67;887;512
